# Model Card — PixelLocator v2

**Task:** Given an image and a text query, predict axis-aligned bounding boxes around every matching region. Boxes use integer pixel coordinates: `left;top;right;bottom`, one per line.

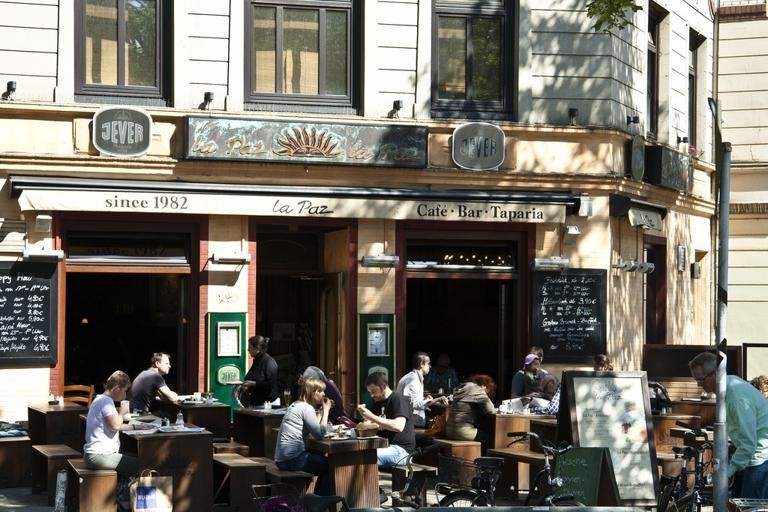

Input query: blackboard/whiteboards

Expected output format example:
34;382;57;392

0;261;58;364
532;269;607;364
554;448;604;507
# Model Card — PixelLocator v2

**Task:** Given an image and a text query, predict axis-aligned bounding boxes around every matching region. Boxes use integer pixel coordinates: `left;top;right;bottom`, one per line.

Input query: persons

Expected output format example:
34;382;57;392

511;347;614;415
689;352;767;511
396;352;498;457
749;376;767;399
84;371;146;512
128;353;178;424
273;365;415;504
241;335;278;410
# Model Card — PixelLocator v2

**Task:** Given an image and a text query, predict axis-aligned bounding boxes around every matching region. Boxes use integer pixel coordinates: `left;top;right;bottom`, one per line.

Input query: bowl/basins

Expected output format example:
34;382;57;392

352;428;380;437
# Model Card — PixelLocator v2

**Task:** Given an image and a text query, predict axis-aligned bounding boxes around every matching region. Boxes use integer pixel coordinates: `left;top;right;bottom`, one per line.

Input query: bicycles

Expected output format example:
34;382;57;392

303;442;439;512
658;419;735;512
431;431;586;507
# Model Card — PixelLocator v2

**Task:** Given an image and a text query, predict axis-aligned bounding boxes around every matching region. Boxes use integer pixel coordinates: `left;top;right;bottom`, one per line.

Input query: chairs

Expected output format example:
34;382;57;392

64;383;94;411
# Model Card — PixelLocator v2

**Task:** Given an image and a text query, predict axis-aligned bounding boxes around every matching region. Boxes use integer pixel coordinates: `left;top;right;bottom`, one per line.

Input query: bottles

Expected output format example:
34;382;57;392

661;405;667;416
165;419;170;429
667;405;673;415
327;422;332;433
177;411;183;427
263;400;272;410
46;391;64;409
206;395;213;405
523;407;530;415
338;425;343;437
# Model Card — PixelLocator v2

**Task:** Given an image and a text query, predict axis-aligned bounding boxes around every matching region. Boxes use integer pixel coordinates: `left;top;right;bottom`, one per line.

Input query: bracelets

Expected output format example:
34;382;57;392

132;424;135;430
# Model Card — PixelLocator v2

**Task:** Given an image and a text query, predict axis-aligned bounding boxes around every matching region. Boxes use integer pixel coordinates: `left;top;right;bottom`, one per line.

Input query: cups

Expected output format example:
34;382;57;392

500;404;507;413
194;392;202;401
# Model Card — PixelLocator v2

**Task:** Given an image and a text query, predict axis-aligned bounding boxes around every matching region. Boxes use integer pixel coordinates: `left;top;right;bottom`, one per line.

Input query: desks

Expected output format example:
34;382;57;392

78;410;213;510
302;430;389;511
29;401;87;456
530;413;702;502
158;398;232;434
234;408;316;454
671;399;715;428
483;410;556;500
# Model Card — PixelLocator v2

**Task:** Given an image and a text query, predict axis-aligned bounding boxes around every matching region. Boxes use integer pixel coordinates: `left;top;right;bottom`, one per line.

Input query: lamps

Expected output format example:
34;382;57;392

676;135;689;147
567;108;578;125
531;256;569;274
356;256;399;276
1;81;17;102
387;100;403;118
562;225;581;246
35;210;51;234
24;250;64;263
202;253;251;277
197;92;213;110
626;114;640;127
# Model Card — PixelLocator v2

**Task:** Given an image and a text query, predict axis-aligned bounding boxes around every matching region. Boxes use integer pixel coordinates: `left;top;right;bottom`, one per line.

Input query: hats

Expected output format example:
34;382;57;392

522;353;543;371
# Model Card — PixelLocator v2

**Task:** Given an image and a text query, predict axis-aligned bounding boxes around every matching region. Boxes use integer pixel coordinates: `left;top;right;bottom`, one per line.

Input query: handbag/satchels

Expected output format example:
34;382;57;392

128;468;172;512
423;415;446;437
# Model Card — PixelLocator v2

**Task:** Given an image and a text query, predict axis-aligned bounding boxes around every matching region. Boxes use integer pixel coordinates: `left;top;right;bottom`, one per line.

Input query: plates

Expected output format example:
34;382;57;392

226;381;244;385
331;436;349;440
356;435;379;440
182;400;203;404
323;433;336;438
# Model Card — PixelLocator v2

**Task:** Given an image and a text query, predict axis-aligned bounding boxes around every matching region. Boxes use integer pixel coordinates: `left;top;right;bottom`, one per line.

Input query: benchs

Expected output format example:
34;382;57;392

412;428;481;486
1;428;31;489
212;452;266;510
655;452;684;481
66;458;117;510
251;457;313;502
487;448;555;498
214;437;250;457
32;444;82;510
